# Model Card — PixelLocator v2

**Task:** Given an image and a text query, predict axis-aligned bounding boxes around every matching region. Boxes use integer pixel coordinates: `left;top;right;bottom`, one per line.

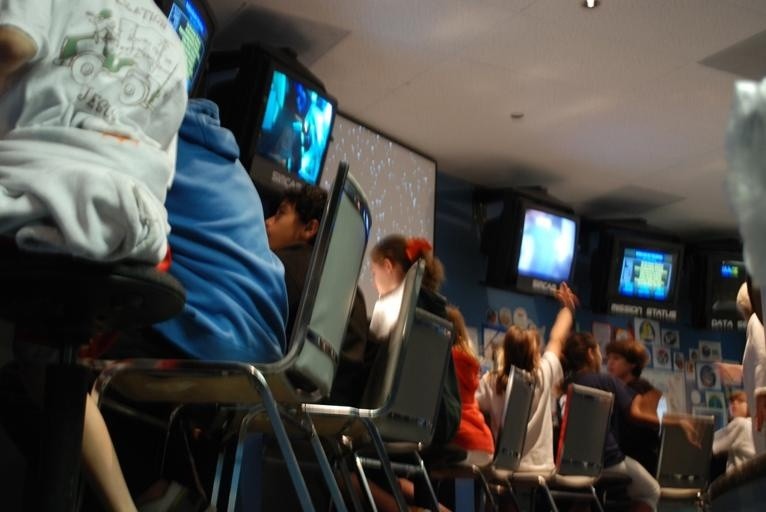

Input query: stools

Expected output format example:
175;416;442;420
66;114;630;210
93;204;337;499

1;248;186;511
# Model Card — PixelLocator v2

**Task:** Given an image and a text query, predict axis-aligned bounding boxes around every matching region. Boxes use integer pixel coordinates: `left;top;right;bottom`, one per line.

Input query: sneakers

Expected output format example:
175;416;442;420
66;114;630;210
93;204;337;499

140;482;189;510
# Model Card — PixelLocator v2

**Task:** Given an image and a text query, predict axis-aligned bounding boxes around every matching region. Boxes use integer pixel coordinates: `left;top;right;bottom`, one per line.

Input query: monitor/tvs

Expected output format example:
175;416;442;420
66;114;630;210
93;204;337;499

154;0;218;99
485;197;580;298
592;227;685;321
206;61;338;187
704;250;749;331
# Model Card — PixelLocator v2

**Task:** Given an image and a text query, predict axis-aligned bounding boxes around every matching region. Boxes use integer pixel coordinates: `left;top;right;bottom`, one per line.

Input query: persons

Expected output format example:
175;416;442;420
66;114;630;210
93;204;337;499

319;284;372;412
0;1;191;512
557;330;703;511
711;388;757;476
83;94;291;511
736;281;766;458
335;231;447;512
600;338;668;511
266;75;313;181
394;297;497;512
139;181;369;512
472;281;578;474
